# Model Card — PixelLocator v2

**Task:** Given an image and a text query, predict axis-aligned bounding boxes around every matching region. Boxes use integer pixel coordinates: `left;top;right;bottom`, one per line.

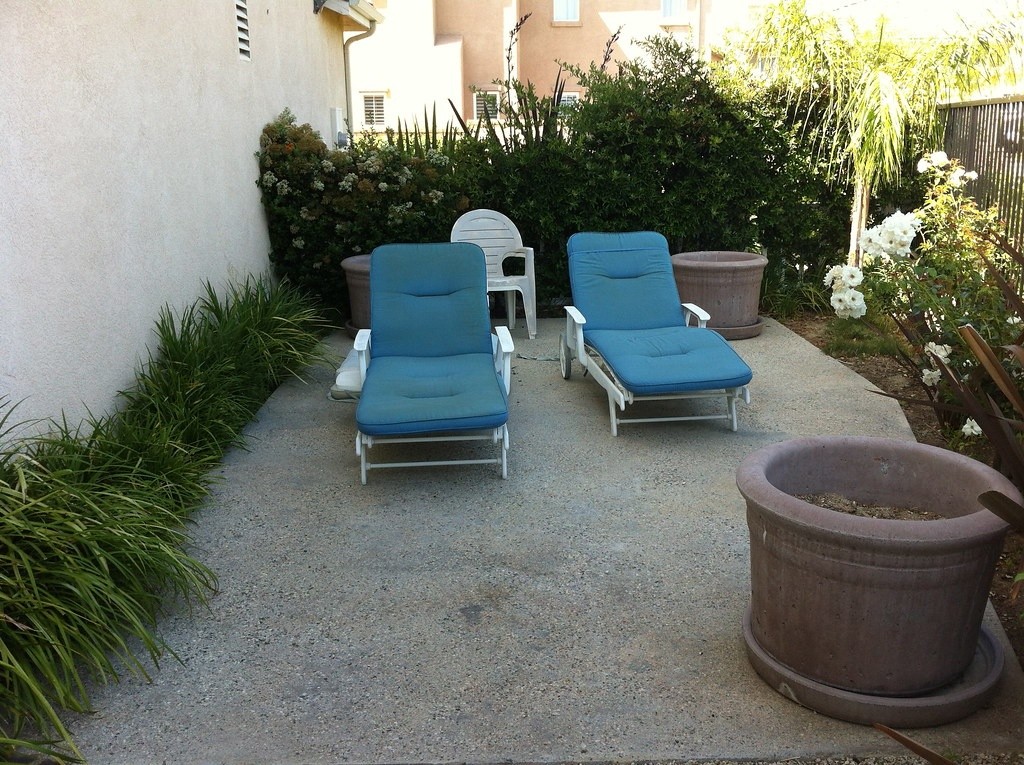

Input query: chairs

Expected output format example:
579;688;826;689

352;242;515;486
449;209;538;340
561;231;754;437
